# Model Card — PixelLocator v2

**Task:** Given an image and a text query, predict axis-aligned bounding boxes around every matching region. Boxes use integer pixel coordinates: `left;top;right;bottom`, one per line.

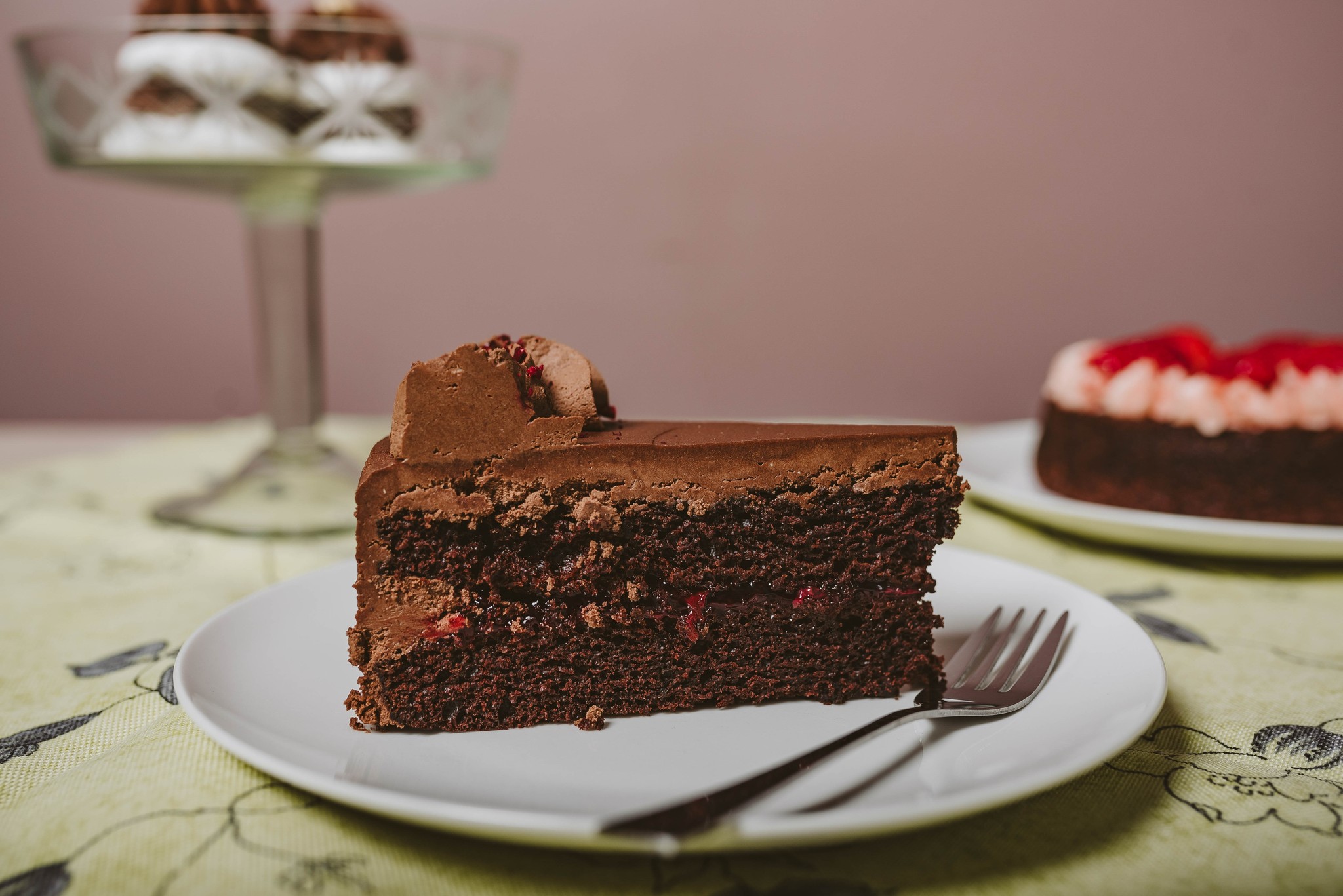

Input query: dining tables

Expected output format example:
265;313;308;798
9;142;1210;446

0;418;1343;896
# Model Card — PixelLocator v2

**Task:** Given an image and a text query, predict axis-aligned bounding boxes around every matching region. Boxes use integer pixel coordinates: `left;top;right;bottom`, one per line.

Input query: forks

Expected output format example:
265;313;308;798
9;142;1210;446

599;603;1068;838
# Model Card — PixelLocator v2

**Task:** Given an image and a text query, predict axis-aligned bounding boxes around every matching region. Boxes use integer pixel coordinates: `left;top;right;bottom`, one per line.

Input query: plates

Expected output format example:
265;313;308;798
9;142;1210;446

955;417;1343;562
170;546;1170;857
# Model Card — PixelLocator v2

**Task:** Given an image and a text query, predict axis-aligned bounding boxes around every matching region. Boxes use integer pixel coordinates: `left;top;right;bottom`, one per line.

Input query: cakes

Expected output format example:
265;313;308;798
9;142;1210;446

346;335;968;735
124;0;417;151
1034;324;1343;527
